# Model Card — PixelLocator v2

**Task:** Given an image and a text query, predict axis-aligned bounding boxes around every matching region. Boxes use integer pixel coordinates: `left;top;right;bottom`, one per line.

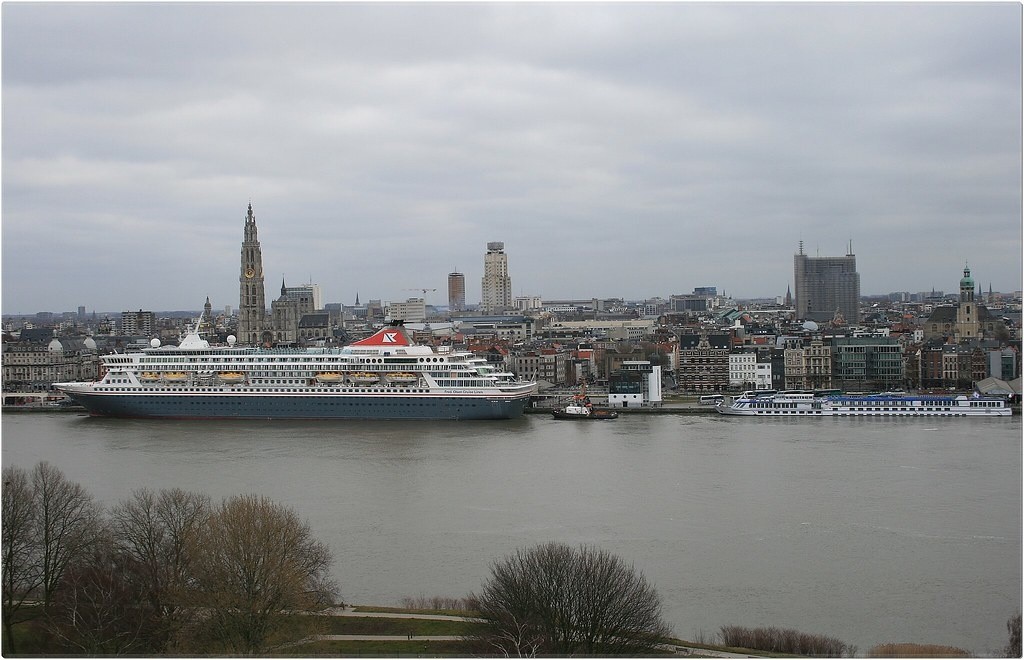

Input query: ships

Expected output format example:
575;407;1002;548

50;311;538;421
715;392;1014;416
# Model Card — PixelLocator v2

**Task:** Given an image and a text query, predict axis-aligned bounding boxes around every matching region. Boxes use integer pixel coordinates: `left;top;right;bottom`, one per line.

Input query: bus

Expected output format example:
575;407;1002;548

697;395;725;405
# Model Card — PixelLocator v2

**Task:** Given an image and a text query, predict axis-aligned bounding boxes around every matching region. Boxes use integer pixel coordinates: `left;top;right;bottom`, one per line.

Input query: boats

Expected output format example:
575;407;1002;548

550;394;619;419
315;374;344;383
349;373;380;383
140;373;159;382
385;373;418;382
218;373;244;384
164;374;189;382
